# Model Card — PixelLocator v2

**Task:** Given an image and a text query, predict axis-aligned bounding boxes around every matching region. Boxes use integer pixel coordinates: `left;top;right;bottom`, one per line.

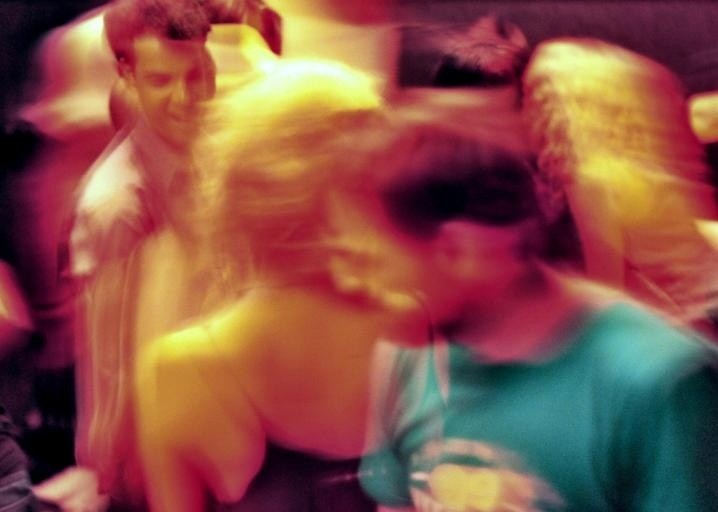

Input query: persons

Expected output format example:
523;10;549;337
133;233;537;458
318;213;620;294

0;265;108;512
327;109;716;511
136;58;426;512
69;2;217;512
